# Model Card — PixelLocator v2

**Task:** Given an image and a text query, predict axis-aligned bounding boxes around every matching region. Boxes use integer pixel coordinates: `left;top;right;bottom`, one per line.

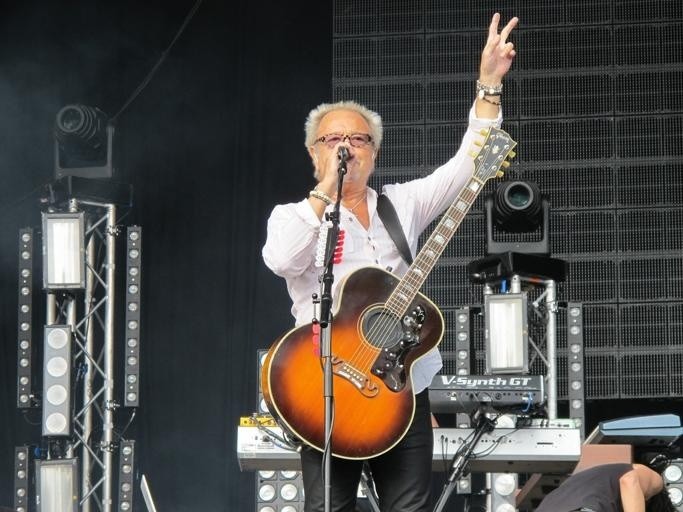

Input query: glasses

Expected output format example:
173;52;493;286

312;131;375;149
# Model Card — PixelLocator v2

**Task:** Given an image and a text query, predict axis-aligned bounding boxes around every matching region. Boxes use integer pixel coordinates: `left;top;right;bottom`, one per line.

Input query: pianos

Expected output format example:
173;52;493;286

236;375;581;473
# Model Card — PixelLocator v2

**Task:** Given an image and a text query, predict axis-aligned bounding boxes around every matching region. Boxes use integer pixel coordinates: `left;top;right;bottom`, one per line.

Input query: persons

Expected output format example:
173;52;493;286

262;11;520;511
533;462;678;512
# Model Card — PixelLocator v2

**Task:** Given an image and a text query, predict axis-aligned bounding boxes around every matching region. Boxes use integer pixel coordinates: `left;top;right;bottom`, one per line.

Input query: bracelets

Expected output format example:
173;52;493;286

483;94;502;105
309;190;332;206
476;79;503;96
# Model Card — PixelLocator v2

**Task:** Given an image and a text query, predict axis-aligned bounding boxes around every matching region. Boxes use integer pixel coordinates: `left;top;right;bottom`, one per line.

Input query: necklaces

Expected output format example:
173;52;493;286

348;192;366;212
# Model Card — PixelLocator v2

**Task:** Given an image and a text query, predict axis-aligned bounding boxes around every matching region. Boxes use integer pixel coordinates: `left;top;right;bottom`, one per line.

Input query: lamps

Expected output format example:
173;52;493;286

52;104;116;200
485;181;551;254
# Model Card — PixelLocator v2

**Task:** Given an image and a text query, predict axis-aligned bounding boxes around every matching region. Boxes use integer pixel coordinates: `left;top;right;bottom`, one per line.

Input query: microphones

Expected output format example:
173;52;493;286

339;148;352;161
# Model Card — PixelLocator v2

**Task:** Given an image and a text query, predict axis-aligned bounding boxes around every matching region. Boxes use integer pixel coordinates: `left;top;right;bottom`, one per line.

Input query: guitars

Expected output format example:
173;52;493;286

262;127;517;461
